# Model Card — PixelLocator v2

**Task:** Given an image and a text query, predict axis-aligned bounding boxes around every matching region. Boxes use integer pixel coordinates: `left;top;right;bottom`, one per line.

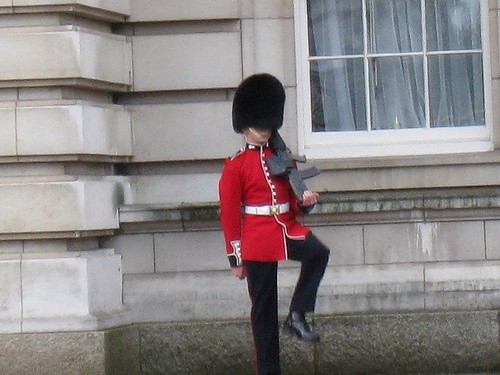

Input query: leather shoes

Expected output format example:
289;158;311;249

282;311;320;344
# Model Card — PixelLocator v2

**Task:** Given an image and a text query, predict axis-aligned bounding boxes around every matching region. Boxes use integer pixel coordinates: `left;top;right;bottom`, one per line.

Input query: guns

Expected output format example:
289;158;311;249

264;128;321;202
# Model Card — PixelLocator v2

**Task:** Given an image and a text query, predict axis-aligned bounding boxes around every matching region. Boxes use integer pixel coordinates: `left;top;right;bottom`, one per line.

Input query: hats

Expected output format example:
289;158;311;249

232;72;286;134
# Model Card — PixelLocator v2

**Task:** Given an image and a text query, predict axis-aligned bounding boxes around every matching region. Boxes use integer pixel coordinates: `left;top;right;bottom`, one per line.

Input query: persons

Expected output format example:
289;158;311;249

218;72;330;375
